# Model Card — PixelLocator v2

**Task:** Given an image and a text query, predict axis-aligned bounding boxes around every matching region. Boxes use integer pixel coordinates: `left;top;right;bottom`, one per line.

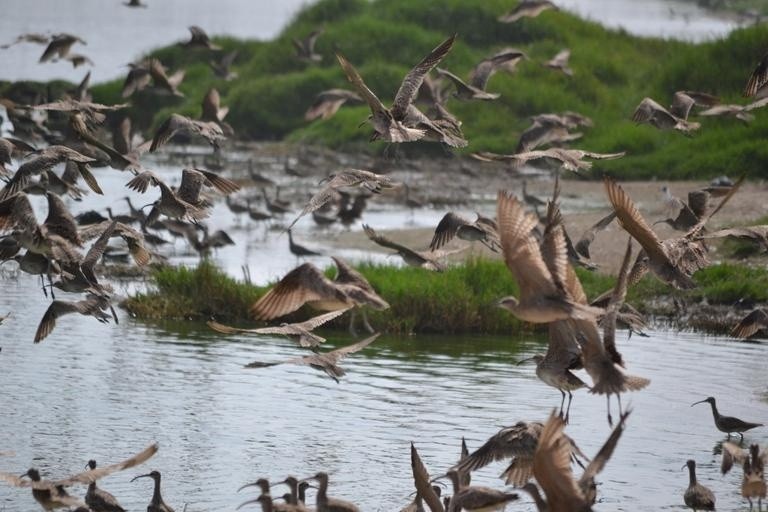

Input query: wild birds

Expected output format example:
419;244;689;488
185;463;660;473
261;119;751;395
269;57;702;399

719;441;768;510
681;459;717;510
234;472;359;510
4;2;768;348
403;402;634;509
691;396;765;443
517;234;652;429
4;440;175;510
